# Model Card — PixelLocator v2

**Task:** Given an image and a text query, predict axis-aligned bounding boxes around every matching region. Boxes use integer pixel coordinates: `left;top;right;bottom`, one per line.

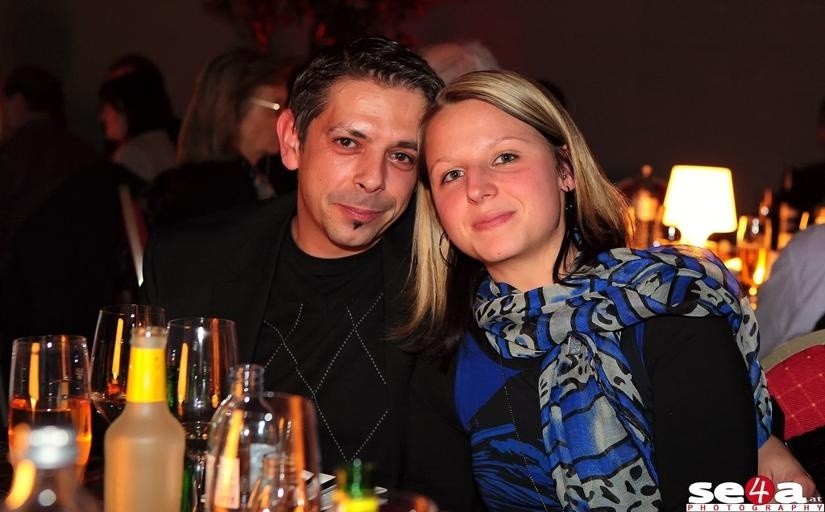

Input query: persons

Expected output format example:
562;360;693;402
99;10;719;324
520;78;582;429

129;35;822;512
399;68;761;512
142;41;295;328
753;223;825;361
98;72;173;285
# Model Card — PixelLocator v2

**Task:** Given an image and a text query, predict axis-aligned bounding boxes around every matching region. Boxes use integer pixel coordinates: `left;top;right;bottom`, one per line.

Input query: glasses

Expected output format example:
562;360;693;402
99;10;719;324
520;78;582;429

245;94;288;115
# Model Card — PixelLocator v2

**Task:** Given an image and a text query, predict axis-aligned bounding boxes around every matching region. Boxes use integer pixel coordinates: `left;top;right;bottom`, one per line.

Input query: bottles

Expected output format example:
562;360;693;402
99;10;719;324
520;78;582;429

102;325;187;512
1;421;99;512
336;459;382;511
204;364;286;512
165;345;198;512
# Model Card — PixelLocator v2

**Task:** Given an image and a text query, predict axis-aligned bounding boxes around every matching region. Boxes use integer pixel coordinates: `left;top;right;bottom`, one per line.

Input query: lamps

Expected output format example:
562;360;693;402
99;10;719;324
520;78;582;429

661;165;738;246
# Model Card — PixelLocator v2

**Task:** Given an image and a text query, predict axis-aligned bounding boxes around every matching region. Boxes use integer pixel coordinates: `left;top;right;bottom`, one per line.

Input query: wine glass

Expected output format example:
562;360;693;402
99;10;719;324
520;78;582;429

9;334;92;493
226;392;325;512
91;306;180;426
166;316;238;512
